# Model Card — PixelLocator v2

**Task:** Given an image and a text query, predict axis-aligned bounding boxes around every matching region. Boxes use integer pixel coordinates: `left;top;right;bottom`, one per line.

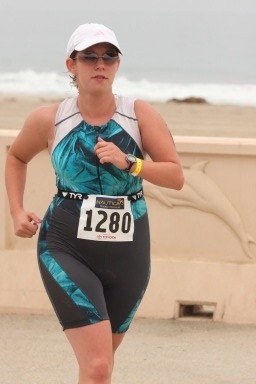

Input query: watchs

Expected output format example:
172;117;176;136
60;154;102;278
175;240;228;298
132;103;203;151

125;154;137;172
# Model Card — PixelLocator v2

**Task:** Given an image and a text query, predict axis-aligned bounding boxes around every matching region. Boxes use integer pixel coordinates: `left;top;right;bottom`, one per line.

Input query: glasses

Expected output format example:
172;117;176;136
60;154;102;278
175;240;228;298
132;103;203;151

73;50;119;66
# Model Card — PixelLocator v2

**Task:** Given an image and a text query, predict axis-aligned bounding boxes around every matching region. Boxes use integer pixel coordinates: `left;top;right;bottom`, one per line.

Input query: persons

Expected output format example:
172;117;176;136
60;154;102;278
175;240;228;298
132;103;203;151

5;23;185;384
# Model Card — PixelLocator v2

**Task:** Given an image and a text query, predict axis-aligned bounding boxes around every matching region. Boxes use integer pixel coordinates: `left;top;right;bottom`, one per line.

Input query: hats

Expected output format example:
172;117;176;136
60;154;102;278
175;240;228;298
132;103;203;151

67;22;123;57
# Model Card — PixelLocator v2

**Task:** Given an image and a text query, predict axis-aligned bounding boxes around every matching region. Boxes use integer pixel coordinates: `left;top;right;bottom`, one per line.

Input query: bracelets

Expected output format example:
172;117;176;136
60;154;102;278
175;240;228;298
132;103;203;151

131;158;142;177
